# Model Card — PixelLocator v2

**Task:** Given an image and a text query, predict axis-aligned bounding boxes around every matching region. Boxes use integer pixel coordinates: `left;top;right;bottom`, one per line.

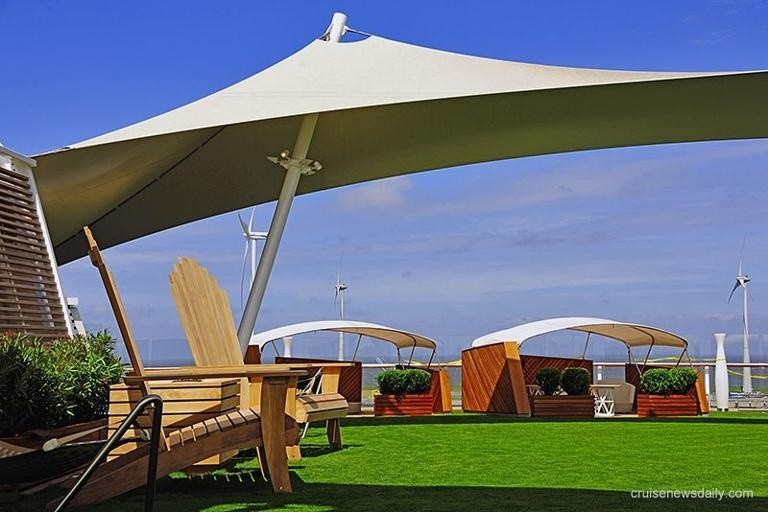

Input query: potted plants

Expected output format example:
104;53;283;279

635;366;699;416
374;369;434;415
0;329;132;463
531;366;596;417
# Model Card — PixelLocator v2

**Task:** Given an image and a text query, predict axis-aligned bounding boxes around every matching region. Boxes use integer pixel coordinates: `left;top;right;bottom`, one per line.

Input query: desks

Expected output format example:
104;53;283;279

524;383;621;418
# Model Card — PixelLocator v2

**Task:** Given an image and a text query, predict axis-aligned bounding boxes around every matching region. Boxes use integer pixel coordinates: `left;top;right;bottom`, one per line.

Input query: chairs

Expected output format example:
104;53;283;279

20;225;354;512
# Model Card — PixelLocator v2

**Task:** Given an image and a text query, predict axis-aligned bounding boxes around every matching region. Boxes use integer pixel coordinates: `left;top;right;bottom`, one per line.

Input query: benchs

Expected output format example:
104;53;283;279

729;392;768;407
